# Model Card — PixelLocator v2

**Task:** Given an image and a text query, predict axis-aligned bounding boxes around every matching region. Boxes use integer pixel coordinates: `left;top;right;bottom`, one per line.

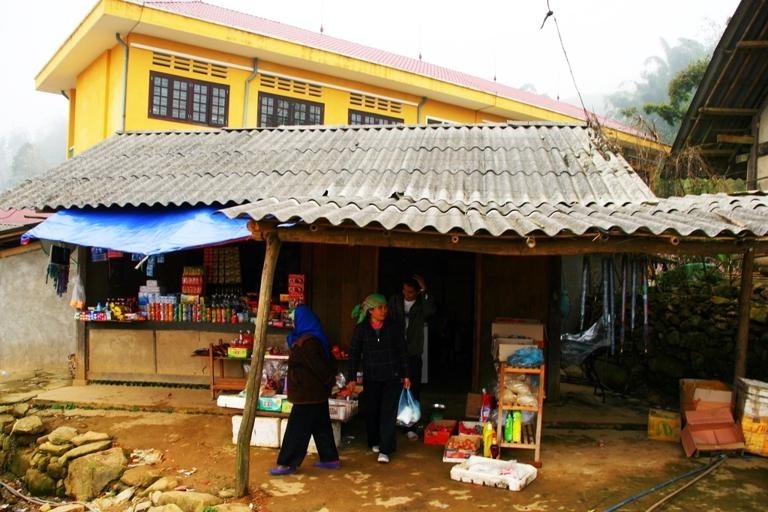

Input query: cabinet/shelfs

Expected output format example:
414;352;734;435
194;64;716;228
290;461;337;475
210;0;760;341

495;361;546;467
208;336;290;400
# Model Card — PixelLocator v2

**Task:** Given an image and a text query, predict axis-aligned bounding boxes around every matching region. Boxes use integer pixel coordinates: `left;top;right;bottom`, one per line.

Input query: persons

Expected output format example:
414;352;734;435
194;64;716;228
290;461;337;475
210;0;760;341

385;270;439;445
268;303;342;476
346;291;412;466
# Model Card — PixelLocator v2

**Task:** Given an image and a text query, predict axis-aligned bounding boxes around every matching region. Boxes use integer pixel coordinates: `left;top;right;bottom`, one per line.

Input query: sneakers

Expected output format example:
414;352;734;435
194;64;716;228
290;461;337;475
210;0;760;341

404;429;419;443
313;460;343;470
372;445;381;455
377;452;391;464
269;464;296;477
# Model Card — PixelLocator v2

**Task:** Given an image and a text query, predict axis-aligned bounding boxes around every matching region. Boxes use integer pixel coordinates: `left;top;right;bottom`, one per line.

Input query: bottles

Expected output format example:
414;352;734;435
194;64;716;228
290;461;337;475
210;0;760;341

146;302;232;322
105;301;109;311
490;432;500;459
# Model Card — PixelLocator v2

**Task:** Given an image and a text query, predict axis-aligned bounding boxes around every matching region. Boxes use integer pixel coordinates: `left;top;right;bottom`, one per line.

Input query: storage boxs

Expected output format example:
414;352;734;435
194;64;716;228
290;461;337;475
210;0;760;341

216;392;359;452
226;347;249;359
177;266;204;304
422;415;489;465
490;337;539;362
464;391;484;420
287;273;307;309
488;314;551;357
647;376;768;459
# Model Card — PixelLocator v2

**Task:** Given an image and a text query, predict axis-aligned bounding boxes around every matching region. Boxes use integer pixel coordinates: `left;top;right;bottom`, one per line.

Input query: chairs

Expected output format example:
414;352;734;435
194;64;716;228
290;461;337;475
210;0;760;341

578;345;634;405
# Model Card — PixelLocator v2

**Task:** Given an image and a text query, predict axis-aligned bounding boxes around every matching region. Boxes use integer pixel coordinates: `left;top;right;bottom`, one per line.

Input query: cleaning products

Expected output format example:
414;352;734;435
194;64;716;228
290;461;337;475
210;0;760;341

479;387;491;424
483;418;499;457
147;302;206;323
